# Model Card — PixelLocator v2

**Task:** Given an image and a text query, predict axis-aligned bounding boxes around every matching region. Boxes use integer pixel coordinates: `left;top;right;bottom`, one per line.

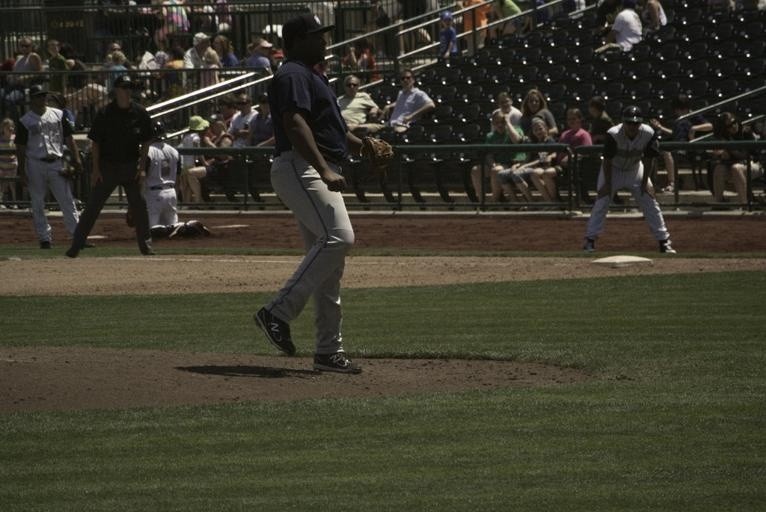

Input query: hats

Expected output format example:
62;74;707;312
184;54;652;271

114;75;135;88
282;12;335;48
153;121;169;142
188;91;269;132
191;32;273;48
28;83;48;96
441;11;453;20
622;106;644;122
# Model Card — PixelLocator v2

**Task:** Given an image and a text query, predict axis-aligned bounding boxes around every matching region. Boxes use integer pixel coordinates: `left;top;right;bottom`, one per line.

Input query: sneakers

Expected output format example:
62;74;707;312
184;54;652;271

40;240;51;249
313;352;360;374
659;240;678;253
475;201;595;214
167;222;217;239
584;240;596;252
137;240;155;254
253;307;295;357
65;239;96;257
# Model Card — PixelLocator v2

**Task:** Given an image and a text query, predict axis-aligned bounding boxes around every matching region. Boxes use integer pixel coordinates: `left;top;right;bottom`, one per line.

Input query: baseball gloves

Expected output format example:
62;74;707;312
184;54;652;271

361;136;393;172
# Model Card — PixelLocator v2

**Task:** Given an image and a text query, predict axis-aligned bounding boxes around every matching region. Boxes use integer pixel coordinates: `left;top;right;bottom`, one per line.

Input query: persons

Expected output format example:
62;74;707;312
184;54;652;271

245;12;396;375
13;84;95;248
582;103;677;256
61;75;160;258
2;2;766;239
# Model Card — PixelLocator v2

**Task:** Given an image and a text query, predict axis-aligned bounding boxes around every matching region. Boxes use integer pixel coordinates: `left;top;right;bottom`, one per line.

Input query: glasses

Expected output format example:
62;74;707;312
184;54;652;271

346;81;359;88
400;76;411;80
